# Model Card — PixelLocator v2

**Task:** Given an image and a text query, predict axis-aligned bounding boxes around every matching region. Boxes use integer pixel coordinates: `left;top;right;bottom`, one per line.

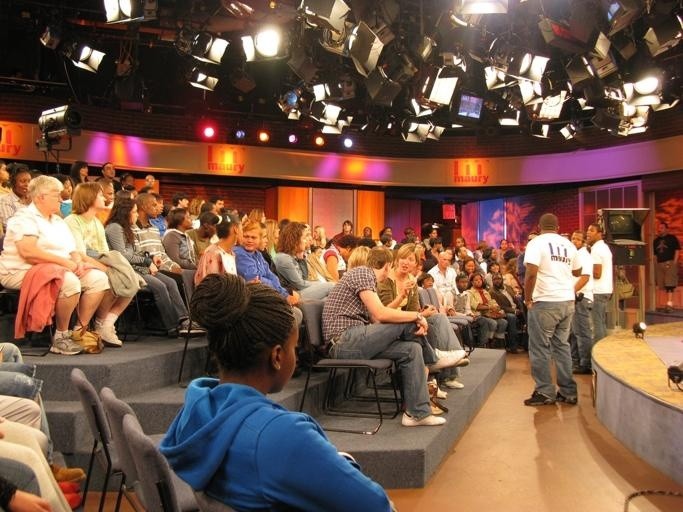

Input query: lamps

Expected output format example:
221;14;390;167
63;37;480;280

486;65;514;88
668;360;683;384
464;1;508;14
313;83;343;101
561;123;577;140
205;127;215;137
237;131;245;139
181;61;219;92
563;51;615;83
508;50;550;82
520;82;545;105
316;136;324;146
419;63;458;105
633;321;647;335
344;138;353;148
241;26;291;62
259;132;269;141
65;36;105;73
104;1;144;22
312;102;341;126
398;110;431;142
623;68;664;105
174;29;231;65
287;107;301;120
40;28;59;48
412;98;436;118
289;134;297;143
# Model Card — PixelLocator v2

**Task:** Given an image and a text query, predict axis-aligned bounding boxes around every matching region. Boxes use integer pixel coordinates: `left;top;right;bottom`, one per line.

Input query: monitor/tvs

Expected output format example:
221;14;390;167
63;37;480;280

608;211;634;234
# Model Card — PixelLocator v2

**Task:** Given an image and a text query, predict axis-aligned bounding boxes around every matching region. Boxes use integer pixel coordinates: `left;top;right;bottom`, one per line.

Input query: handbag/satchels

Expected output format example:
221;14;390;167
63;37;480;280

71;325;105;355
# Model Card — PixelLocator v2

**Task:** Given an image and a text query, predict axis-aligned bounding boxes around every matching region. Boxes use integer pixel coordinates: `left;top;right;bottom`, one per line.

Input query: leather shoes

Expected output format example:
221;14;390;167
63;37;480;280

50;463;88;484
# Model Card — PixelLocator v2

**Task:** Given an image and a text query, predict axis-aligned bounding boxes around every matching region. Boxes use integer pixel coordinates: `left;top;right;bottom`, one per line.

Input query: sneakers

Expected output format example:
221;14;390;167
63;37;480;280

429;404;444;415
556;391;578;405
178;319;205;338
455;359;471;367
402;412;446;427
524;392;556;406
442;380;465;390
50;338;83;355
426;347;467;370
95;324;122;348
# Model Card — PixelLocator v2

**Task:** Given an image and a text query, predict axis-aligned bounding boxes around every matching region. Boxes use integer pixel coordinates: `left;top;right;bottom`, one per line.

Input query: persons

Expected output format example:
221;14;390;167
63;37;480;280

52;173;74;218
210;194;224;215
421;238;444;272
135;194;191;316
158;273;394;512
444;275;488;348
444;246;461;274
322;246;466;427
230;213;233;214
169;190;191;210
94;162;122;194
359;225;372;240
194;213;243;285
420;229;447;252
374;226;397;250
347;246;371;271
149;193;169;237
264;220;280;259
0;175;110;356
472;240;488;263
248;208;266;228
479;246;500;274
162;208;200;270
489;273;527;354
495;239;515;264
392;227;418;249
587;223;614;341
483;261;506;286
312;225;329;250
70;160;89;188
0;161;12;197
523;213;582;406
220;207;239;218
465;273;508;349
300;222;336;283
185;212;221;264
320;234;359;282
517;233;540;324
454;246;474;272
389;243;470;389
63;183;146;347
567;230;594;374
105;197;208;337
653;222;682;312
233;218;303;378
502;258;524;295
454;236;474;260
416;273;463;351
0;167;31;255
239;210;248;225
327;218;354;246
459;257;477;274
1;342;87;512
118;174;135;192
138;174;156;193
428;252;458;296
96;178;118;226
192;202;218;230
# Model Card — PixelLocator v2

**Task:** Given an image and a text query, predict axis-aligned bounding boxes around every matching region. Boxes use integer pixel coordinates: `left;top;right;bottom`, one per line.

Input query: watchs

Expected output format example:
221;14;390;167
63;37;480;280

523;299;532;305
416;311;424;321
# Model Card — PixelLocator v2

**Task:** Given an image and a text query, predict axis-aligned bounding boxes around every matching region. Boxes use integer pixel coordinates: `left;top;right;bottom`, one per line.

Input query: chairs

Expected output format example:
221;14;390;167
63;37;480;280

299;300;399;434
122;413;180;511
70;369;167;512
177;269;211;388
100;387;197;512
